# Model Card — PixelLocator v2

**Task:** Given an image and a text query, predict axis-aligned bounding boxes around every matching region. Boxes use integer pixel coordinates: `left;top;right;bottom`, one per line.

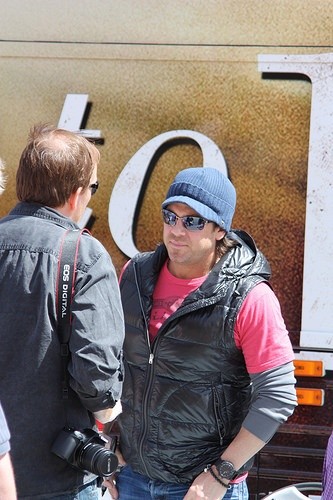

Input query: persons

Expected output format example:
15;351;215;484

0;128;125;500
0;406;18;500
98;167;298;500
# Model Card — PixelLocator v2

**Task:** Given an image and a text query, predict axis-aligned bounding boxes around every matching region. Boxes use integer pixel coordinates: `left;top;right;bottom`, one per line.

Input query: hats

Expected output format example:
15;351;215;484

161;167;236;233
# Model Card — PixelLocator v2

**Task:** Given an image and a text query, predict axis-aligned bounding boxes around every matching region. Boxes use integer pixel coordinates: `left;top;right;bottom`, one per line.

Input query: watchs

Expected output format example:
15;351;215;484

214;458;238;480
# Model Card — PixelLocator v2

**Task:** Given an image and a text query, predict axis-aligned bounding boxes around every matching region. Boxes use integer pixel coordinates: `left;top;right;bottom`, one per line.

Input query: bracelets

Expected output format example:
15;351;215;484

208;466;230;488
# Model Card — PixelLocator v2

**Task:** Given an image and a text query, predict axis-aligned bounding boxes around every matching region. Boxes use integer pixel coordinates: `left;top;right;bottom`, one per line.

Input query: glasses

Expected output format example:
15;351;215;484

162;209;212;231
89;182;98;195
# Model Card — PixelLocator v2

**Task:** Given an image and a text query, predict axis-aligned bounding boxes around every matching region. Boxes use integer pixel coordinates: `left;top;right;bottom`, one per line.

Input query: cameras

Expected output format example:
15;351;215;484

50;425;119;477
